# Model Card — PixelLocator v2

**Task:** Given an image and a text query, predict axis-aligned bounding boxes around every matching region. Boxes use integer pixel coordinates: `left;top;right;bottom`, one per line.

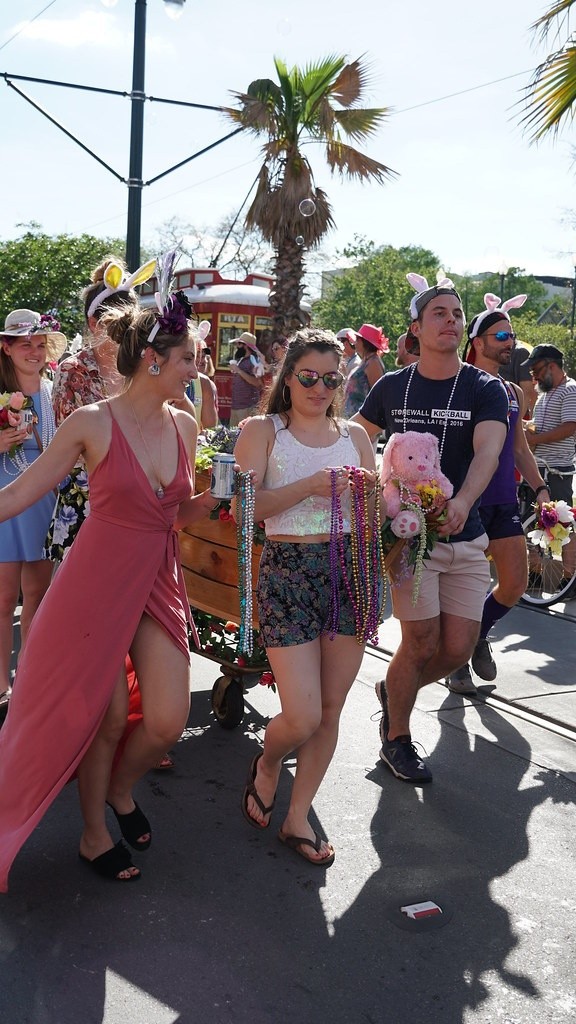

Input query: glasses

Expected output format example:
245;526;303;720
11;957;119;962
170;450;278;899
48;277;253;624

529;360;556;376
477;332;516;340
272;346;283;352
286;365;344;390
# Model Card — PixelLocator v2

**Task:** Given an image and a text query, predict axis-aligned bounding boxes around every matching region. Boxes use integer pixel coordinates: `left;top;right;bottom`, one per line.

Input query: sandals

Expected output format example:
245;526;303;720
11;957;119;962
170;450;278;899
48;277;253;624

151;753;176;769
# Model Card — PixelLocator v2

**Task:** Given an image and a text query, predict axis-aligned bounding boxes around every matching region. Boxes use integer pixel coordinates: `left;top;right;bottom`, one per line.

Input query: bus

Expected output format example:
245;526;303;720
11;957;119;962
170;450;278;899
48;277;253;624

133;149;313;427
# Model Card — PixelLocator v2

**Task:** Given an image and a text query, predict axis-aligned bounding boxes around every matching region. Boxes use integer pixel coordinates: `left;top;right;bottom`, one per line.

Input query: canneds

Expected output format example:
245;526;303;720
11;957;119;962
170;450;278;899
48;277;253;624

16;409;33;441
209;452;236;501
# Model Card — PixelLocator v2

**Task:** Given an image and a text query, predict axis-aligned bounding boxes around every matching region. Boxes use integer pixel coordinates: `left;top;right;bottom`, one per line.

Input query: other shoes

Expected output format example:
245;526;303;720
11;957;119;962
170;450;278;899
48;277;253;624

0;691;11;709
526;570;544;591
551;578;576;602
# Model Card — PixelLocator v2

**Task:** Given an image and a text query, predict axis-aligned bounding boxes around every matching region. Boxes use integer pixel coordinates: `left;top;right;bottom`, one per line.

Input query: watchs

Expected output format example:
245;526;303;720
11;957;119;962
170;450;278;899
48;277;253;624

536;485;551;496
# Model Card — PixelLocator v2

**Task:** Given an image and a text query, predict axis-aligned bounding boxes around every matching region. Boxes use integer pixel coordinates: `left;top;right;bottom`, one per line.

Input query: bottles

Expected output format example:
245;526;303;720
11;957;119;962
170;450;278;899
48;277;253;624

524;421;536;452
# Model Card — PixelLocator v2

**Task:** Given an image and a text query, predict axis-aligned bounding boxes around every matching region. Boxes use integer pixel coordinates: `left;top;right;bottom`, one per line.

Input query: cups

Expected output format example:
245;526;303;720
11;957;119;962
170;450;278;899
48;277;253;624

230;359;237;373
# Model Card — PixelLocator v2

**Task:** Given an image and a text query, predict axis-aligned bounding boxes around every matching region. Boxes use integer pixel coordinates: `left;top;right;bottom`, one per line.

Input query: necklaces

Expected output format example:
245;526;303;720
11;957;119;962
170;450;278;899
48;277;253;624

320;464;387;647
403;359;461;459
2;377;57;475
126;391;164;498
236;468;254;658
231;357;244;374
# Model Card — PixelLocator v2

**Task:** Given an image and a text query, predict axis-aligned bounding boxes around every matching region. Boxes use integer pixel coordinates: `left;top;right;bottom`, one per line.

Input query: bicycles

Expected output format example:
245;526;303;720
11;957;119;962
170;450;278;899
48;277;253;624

515;456;576;609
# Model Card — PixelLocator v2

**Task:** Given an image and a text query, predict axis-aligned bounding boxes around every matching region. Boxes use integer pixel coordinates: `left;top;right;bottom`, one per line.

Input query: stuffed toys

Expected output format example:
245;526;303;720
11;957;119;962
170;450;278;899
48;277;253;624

379;431;454;519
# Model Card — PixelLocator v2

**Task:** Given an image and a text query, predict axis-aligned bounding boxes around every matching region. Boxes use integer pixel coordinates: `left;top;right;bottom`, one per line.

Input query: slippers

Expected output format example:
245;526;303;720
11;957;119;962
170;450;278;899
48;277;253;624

104;800;152;852
241;752;277;831
79;843;143;882
278;826;335;864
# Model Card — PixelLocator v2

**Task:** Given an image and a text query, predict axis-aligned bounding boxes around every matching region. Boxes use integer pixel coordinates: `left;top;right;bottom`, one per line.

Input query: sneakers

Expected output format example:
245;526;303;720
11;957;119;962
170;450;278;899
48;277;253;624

378;731;433;782
370;680;389;744
445;663;477;695
472;638;496;680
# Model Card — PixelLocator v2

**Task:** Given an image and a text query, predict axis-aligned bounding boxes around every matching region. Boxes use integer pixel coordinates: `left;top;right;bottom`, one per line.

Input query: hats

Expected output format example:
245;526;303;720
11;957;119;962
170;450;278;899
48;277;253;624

463;311;509;365
355;324;389;350
336;328;356;344
0;309;68;362
520;345;563;368
200;340;211;356
229;332;258;353
405;285;462;356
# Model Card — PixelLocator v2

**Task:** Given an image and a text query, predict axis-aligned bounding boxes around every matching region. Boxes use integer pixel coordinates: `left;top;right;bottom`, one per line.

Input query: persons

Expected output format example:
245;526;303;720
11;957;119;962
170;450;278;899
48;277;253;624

0;309;67;706
0;290;257;892
345;323;390;454
520;344;576;597
168;320;218;429
335;328;362;374
395;333;420;367
498;343;534;418
233;329;387;864
444;293;551;694
345;273;510;783
228;332;264;428
272;338;287;362
42;260;156;586
